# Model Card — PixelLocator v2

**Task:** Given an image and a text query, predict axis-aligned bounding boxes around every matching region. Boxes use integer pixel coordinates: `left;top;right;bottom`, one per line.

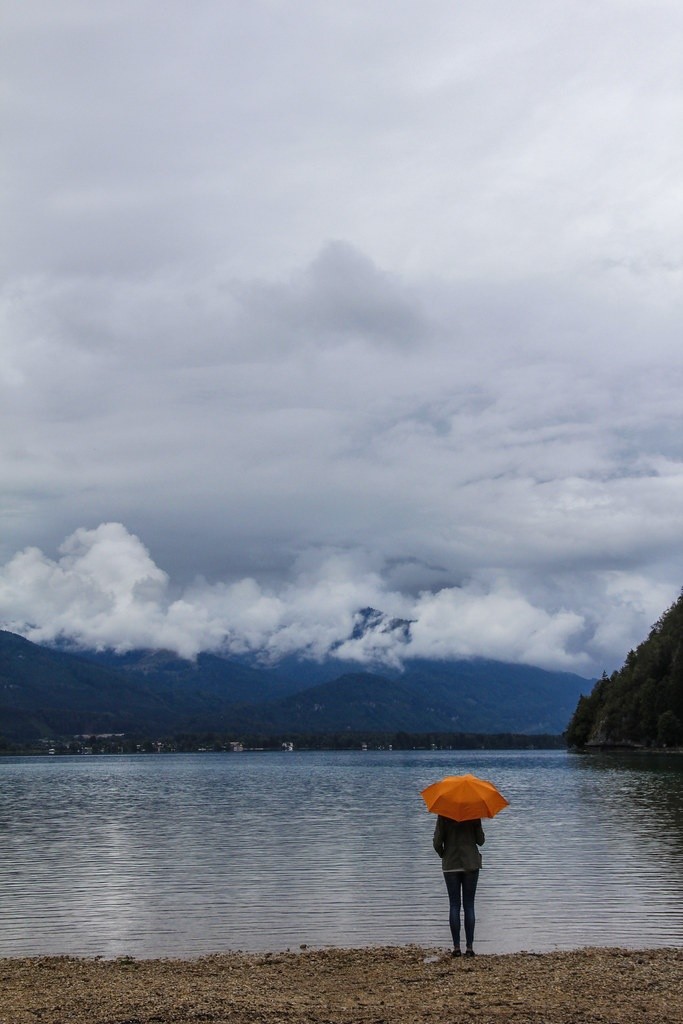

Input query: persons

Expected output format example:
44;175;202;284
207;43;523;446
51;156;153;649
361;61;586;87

433;814;485;956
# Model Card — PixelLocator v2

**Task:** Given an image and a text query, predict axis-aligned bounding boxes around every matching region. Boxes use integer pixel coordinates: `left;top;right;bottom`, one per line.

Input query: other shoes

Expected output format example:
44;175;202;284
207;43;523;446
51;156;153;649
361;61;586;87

452;949;475;956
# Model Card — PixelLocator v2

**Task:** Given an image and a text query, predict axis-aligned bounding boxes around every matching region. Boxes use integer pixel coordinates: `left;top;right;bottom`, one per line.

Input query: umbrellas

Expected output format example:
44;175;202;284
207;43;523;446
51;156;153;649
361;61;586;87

420;774;510;823
